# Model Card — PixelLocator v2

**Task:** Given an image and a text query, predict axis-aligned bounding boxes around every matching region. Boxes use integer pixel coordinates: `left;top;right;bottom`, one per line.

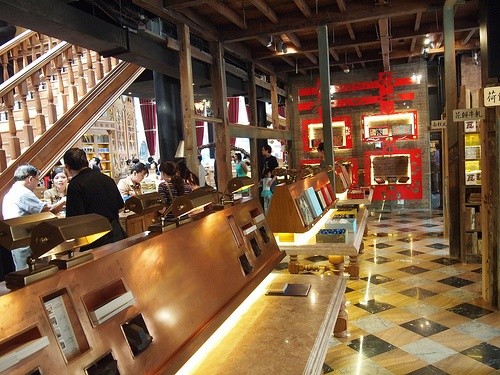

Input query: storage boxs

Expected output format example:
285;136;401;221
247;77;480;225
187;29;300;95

315;203;359;243
347;186;371;199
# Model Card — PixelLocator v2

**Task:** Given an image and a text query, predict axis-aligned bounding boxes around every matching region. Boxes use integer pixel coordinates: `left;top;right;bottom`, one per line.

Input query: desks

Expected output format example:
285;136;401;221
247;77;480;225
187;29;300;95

178;274;349;374
334;187;375;211
274;209;362;281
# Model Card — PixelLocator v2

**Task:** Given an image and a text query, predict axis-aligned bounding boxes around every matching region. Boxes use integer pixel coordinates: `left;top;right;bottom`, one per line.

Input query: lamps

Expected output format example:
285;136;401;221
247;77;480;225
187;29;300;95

123;176;257;231
267;34;287;55
272;165;323;184
0;209;113;294
196;96;213;114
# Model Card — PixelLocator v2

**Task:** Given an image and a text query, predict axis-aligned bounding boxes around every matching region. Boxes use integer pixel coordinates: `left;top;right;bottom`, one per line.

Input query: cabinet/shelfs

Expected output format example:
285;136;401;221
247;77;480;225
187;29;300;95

74;127;113;177
461;120;484;263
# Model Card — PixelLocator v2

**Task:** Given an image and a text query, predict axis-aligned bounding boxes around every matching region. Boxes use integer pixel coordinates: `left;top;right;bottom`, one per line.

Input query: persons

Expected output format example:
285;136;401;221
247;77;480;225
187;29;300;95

43;144;278;208
431;143;441;192
2;164;67;272
64;147;129;252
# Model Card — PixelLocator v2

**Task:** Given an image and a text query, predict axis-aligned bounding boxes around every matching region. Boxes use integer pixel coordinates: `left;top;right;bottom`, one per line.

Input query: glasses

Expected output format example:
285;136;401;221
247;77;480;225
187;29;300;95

28;175;41;184
